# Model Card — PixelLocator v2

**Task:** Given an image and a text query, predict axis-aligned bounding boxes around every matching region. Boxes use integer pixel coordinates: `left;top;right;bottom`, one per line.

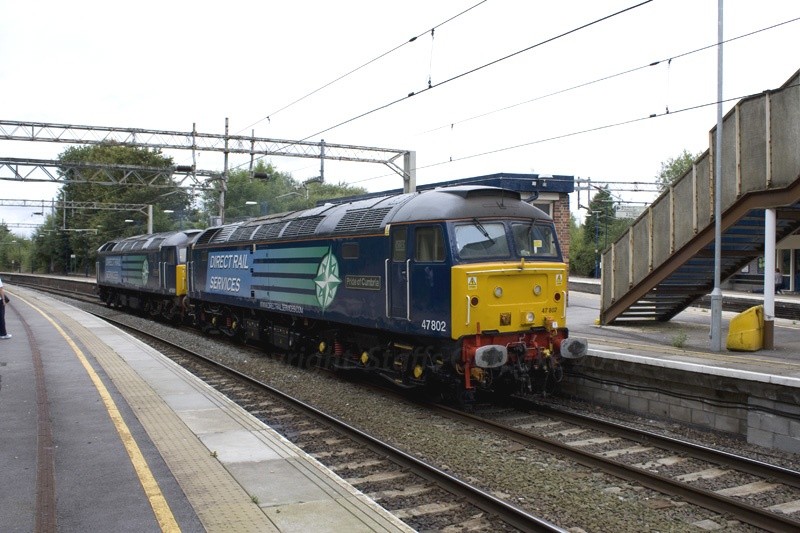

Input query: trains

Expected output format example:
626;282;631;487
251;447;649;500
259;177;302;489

98;185;572;411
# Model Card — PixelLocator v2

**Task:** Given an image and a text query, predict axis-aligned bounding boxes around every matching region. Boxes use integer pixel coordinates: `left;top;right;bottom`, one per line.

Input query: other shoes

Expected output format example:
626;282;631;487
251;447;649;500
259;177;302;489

1;334;12;339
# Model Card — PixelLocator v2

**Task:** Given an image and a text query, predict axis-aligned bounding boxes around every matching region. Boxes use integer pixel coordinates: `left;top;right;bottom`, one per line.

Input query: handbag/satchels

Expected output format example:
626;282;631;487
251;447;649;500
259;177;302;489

3;294;10;305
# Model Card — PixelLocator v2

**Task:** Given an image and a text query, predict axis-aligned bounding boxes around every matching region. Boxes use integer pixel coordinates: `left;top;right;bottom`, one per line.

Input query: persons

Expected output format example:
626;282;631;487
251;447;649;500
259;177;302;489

0;277;12;339
776;268;784;293
